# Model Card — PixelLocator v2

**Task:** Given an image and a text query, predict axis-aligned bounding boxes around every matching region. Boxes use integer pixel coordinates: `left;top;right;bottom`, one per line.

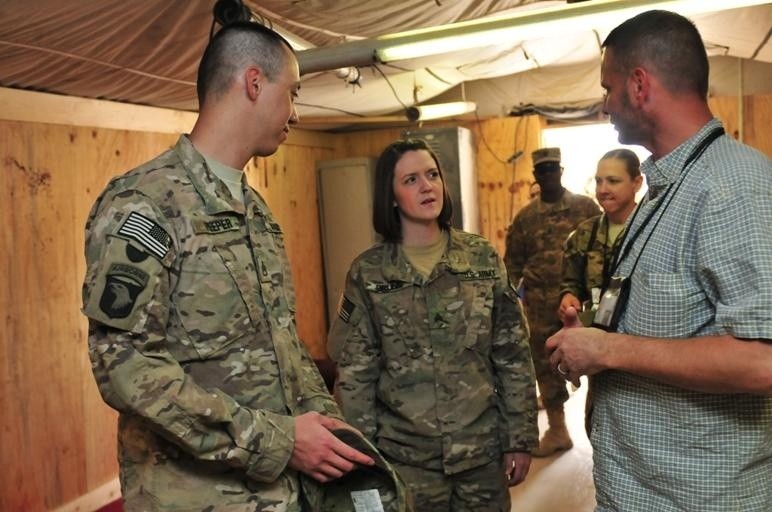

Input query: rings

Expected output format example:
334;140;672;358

556;364;568;376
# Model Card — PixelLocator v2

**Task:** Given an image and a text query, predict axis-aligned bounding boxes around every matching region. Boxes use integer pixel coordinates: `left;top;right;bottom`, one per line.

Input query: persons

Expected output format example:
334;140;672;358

325;138;538;512
556;149;644;326
543;9;772;512
503;148;602;457
81;20;412;512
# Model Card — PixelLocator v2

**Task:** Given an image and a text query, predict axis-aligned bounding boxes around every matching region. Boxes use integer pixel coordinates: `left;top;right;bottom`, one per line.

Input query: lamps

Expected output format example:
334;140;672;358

404;82;477;122
212;0;364;93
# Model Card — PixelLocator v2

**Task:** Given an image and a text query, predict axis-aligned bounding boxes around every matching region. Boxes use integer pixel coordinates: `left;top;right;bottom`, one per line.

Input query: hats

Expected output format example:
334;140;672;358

531;148;561;167
299;428;415;512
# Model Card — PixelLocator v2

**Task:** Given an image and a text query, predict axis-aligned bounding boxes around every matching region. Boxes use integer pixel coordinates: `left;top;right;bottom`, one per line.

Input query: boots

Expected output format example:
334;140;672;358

530;406;572;458
